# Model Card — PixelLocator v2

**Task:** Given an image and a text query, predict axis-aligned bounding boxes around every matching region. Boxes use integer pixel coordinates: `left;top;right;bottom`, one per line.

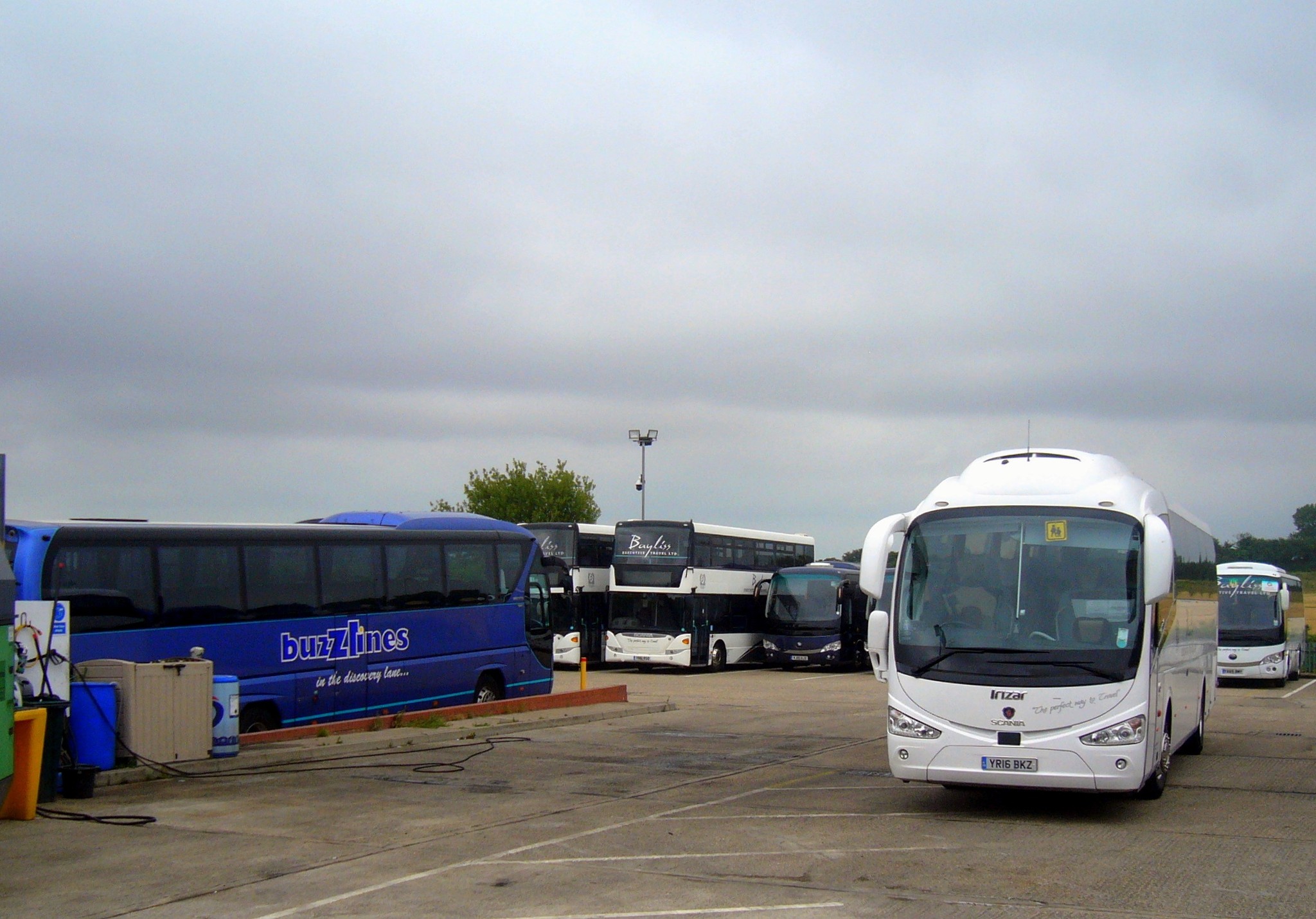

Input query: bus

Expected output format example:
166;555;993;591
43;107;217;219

1217;562;1306;687
516;521;618;664
0;509;575;731
605;518;818;673
752;560;897;670
858;446;1220;795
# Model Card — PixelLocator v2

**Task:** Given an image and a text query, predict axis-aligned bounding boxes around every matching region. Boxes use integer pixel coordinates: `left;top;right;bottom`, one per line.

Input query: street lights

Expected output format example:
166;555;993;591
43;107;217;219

628;427;659;521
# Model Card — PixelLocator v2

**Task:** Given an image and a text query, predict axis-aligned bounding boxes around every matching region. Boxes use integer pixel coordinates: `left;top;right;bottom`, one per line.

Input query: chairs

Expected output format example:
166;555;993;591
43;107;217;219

1073;617;1111;643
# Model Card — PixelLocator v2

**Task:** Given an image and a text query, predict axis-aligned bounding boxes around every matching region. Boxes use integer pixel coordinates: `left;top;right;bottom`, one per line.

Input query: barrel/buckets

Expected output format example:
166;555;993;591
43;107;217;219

61;764;99;797
66;682;117;770
210;675;240;758
